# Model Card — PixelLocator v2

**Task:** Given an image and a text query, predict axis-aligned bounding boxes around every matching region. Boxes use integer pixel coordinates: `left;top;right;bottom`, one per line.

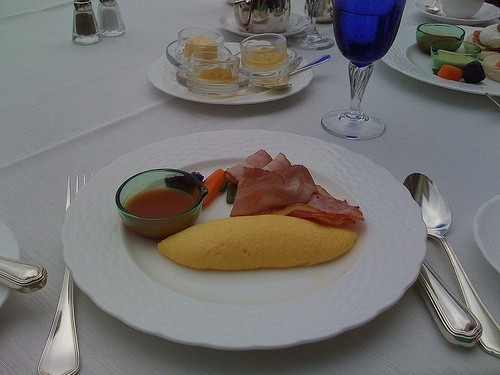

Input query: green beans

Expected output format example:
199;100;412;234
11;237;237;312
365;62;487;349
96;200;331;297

220;180;236;203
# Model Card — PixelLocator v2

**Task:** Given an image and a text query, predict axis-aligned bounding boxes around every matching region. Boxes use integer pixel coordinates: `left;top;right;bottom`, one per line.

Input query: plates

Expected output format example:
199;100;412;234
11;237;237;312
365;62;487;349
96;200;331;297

147;45;315;105
219;12;312;37
379;19;500;97
412;0;500;26
472;192;500;274
0;223;20;305
61;131;425;348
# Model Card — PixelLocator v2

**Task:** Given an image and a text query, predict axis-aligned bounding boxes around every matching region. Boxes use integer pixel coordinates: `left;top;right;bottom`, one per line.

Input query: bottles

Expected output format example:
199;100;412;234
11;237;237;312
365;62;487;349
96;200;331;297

69;0;103;45
97;0;126;37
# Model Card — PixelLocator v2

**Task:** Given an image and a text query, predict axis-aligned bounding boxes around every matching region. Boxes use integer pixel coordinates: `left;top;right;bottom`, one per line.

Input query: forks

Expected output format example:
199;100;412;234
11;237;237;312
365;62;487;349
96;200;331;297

37;173;94;375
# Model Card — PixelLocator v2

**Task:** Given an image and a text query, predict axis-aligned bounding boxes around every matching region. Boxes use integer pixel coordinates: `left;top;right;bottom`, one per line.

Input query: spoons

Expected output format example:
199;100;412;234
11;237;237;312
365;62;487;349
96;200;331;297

401;173;500;359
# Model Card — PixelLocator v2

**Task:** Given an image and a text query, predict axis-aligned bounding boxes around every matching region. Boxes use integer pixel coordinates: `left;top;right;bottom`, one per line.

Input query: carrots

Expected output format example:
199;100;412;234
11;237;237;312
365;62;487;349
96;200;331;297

203;168;225;206
437;64;462;81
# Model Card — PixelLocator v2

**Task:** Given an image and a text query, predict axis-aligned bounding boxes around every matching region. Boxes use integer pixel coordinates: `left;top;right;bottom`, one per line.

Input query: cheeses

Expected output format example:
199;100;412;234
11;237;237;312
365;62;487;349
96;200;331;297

183;36;218;60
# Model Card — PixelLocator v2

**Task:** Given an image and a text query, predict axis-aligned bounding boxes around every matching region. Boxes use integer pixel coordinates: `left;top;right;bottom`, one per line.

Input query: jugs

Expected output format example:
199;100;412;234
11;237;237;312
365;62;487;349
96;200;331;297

228;0;334;34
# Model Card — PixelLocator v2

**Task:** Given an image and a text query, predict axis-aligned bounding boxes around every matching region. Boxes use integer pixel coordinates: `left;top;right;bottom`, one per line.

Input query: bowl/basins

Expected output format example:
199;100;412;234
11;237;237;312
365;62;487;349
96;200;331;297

166;25;299;92
415;21;482;74
437;0;485;18
114;168;209;240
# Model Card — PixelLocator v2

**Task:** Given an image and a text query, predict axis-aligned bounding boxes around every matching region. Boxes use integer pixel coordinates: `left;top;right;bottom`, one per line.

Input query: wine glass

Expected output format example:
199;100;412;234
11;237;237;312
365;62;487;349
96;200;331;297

321;0;408;140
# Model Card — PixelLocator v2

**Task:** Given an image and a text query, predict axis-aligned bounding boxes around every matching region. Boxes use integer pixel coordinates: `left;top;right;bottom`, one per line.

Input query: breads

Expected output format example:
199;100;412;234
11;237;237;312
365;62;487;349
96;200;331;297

465;24;500;81
157;215;359;271
